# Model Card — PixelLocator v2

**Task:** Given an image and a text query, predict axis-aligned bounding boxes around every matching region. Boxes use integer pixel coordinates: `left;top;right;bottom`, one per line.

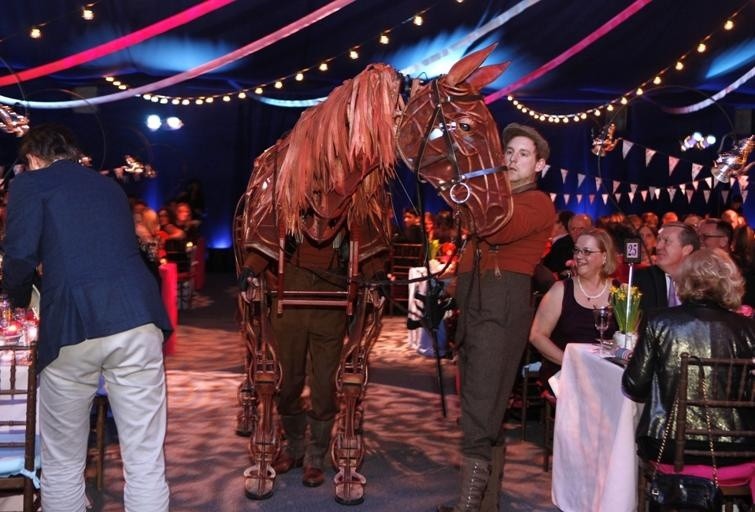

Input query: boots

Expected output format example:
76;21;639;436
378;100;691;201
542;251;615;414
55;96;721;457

452;446;505;512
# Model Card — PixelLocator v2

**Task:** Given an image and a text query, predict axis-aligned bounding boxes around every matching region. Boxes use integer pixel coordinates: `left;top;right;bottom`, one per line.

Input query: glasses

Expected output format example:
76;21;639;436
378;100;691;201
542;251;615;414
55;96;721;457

572;247;603;256
698;233;724;242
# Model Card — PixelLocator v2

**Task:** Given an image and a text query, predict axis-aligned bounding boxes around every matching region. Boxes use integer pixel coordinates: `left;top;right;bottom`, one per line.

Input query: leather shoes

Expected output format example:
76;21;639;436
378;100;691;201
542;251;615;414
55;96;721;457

272;446;296;476
302;463;324;488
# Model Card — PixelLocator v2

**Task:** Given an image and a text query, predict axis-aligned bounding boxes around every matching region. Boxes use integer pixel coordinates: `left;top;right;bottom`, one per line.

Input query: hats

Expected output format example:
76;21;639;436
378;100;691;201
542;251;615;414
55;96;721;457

502;121;551;164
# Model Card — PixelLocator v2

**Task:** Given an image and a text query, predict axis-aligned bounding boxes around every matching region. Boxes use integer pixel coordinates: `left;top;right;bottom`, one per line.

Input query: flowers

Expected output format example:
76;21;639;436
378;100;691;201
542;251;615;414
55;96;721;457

605;280;641;334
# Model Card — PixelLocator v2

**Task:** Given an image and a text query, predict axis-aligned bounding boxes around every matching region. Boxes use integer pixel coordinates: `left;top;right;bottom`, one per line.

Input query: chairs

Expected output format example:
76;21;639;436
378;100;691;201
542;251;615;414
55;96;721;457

636;351;755;512
523;337;569;471
149;214;216;362
0;340;108;512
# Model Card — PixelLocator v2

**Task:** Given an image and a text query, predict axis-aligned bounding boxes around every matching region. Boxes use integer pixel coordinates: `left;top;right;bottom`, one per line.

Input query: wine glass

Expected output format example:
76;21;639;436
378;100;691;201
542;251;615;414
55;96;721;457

594;304;611;352
0;302;36;363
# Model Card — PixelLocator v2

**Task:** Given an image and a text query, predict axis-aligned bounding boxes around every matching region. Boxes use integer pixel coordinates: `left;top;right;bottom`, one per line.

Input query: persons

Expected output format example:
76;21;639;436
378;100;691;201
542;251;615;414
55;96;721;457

401;121;557;512
238;234;396;489
3;121;175;512
402;207;458;244
129;196;201;296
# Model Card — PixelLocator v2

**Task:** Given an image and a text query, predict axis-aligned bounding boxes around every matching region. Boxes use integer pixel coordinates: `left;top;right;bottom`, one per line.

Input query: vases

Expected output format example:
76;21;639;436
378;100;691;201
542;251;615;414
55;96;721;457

612;328;639;348
